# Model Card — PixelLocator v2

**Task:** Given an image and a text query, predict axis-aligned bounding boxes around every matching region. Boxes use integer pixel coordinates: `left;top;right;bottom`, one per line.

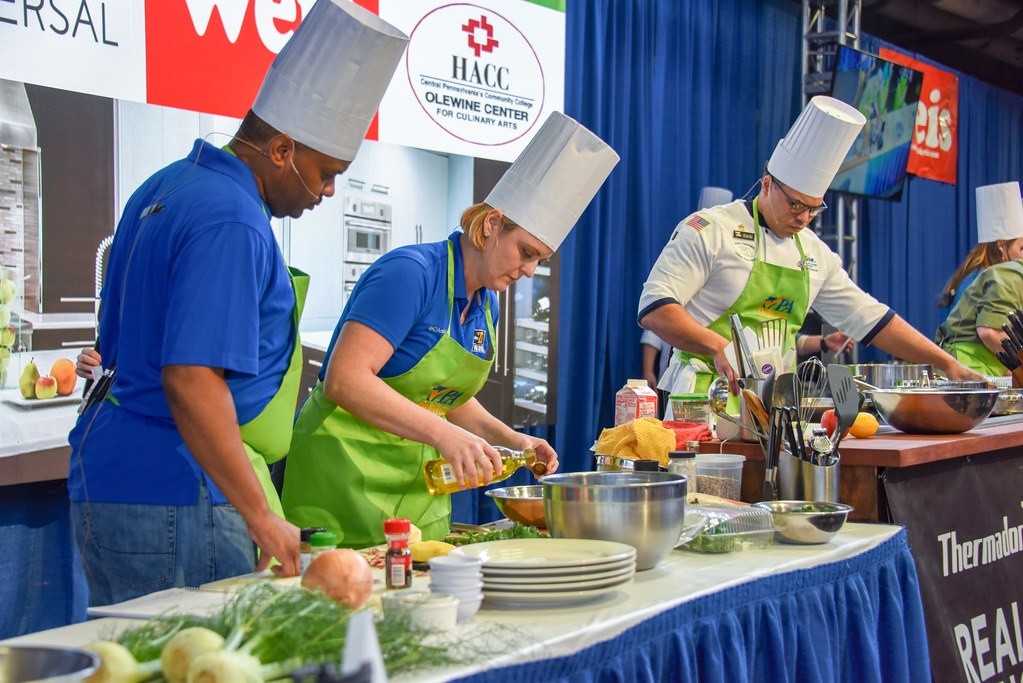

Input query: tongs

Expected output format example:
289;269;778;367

764;405;806;501
729;312;760;380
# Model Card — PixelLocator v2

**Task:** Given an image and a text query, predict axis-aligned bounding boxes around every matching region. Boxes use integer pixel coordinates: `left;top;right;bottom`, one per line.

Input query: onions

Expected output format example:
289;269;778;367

300;548;372;609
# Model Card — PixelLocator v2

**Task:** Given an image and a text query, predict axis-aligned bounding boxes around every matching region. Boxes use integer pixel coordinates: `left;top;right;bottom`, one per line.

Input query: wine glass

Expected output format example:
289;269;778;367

667;451;697;494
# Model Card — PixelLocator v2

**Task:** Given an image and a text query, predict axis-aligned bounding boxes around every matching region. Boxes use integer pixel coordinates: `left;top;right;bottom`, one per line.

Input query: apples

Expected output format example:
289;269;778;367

35;374;58;399
0;275;16;388
820;410;851;440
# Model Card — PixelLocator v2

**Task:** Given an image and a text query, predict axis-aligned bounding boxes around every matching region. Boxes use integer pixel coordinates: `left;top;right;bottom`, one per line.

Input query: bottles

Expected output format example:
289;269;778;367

422;446;537;496
300;526;327;575
384;518;413;590
309;532;337;563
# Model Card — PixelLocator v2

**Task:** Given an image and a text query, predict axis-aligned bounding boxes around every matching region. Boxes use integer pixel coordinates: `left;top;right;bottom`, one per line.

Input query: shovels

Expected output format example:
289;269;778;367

828;364;859;463
754;318;787;357
773;373;802;433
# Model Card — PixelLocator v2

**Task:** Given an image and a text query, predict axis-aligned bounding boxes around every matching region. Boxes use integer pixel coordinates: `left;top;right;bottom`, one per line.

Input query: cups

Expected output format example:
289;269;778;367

716;415;741;442
777;450;840;503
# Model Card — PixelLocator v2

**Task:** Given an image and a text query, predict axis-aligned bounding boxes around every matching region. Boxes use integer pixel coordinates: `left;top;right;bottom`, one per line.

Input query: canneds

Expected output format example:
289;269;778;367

299;526;338;577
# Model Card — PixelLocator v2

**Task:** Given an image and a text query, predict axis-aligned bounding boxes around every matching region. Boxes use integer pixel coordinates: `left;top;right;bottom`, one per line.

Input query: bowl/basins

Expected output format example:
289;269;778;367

667;393;710;425
801;398;835;420
484;485;547;530
751;501;854;545
399;592;459;633
694;454;747;503
538;471;688;571
381;591;422;622
427;555;485;623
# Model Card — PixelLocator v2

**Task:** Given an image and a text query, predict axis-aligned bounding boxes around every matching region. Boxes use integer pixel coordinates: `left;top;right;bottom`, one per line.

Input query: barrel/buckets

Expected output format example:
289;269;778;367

855;363;933;388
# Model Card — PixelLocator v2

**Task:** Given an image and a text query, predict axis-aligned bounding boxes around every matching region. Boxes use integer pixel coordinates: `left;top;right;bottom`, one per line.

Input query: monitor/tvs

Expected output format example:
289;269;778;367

830;43;924;202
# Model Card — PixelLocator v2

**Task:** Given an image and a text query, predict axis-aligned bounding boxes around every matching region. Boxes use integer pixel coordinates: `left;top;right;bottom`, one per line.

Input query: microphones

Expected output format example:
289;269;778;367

289;156;319;198
768;189;779;225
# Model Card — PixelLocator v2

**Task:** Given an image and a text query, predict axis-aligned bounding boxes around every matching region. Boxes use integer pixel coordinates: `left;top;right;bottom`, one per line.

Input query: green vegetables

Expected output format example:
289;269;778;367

790;506;835;513
445;522;551;546
680;521;745;554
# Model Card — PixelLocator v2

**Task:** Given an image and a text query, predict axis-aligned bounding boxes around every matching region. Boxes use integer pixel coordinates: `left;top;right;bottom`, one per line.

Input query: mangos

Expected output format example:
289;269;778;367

50;358;78;395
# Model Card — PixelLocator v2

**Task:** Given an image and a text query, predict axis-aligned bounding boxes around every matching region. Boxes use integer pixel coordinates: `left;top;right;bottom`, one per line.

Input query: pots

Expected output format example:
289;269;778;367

861;388;1005;435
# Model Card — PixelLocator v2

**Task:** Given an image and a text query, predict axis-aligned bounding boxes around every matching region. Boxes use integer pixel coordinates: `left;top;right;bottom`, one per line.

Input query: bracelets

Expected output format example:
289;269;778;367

821;334;829;355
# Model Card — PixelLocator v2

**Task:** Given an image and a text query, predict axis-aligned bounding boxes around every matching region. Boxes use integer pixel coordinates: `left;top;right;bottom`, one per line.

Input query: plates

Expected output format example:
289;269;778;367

448;538;637;608
2;388;82;406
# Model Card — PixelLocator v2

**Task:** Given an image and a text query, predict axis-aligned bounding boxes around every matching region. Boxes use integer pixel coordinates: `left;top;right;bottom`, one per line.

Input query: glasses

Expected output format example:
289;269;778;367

771;179;829;218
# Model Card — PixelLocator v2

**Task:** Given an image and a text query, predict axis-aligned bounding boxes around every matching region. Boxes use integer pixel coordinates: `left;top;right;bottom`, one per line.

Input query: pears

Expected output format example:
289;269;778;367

19;357;40;399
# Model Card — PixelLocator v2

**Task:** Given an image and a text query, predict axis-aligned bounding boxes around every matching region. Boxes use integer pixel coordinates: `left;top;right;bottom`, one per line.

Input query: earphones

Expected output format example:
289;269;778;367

999;247;1003;251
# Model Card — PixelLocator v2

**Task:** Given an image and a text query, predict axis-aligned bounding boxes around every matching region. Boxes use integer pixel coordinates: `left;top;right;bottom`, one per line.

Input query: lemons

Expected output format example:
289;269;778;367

848;412;879;439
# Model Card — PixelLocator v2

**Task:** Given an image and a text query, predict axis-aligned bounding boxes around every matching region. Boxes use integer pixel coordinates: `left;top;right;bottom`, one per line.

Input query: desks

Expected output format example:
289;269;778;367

838;101;918;196
700;414;1023;683
0;348;91;487
0;517;933;683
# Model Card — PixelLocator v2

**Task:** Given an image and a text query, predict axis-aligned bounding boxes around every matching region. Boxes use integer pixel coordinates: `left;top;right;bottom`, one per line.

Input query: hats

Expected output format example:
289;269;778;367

975;182;1023;244
767;95;866;198
251;0;411;162
483;111;620;253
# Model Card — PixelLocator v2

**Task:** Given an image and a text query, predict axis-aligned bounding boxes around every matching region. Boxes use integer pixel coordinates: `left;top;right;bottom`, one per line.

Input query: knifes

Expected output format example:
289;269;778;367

995;308;1023;371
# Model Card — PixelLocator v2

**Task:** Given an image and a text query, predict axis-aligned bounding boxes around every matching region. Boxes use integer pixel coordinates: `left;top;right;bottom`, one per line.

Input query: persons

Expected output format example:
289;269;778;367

637;95;988;422
282;110;619;546
65;1;409;617
936;181;1022;378
